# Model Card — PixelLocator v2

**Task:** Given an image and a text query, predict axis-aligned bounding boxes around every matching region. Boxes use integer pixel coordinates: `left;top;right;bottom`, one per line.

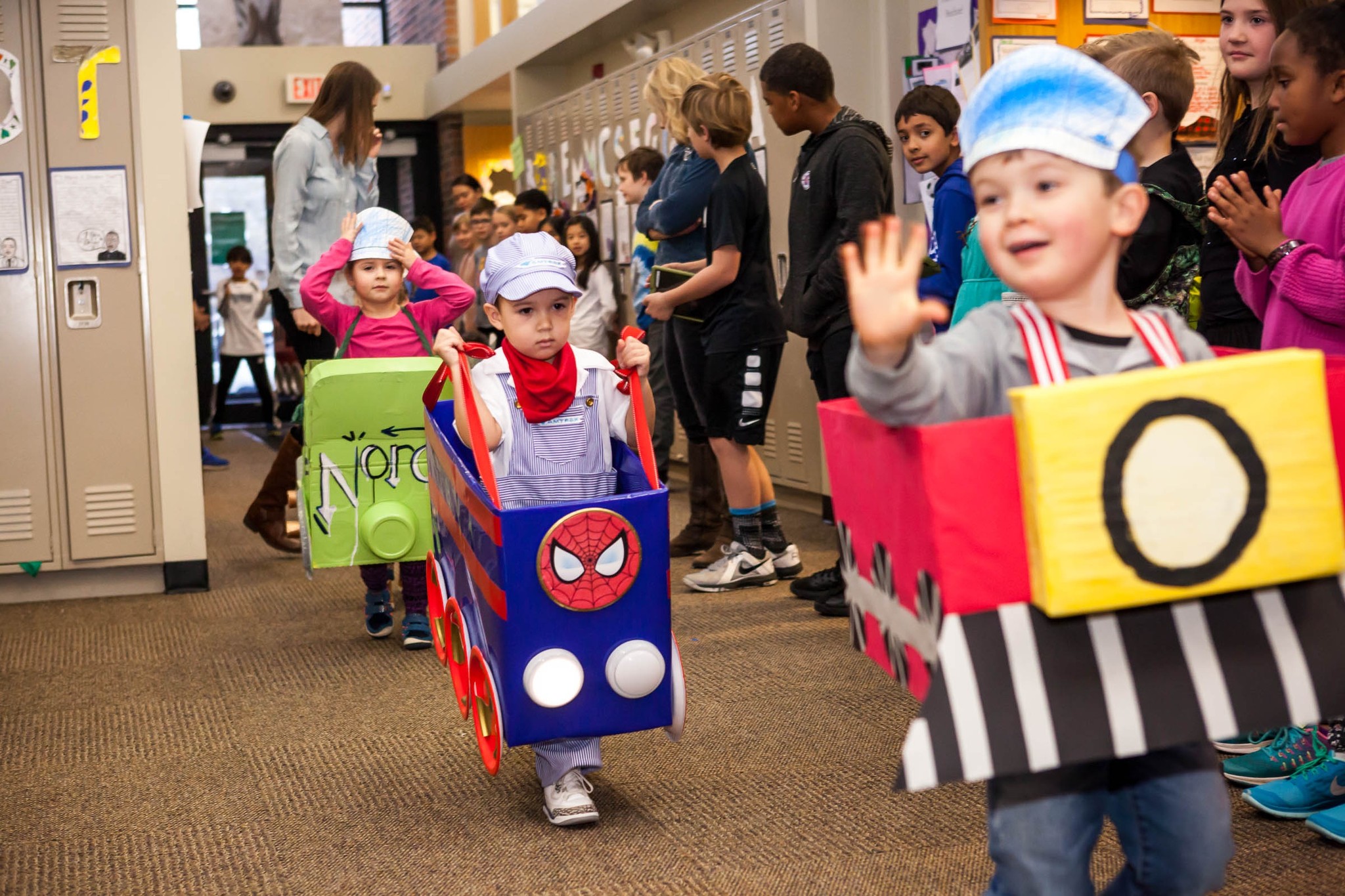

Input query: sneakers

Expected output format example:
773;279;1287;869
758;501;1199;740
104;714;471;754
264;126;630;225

540;767;600;826
789;562;847;599
1241;750;1345;818
1213;723;1288;754
1219;724;1330;784
681;541;778;591
765;540;803;577
815;596;851;616
1304;803;1345;844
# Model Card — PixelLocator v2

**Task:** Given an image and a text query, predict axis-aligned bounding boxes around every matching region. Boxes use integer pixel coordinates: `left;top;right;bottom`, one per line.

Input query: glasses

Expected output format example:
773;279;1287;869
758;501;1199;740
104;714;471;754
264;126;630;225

470;218;492;226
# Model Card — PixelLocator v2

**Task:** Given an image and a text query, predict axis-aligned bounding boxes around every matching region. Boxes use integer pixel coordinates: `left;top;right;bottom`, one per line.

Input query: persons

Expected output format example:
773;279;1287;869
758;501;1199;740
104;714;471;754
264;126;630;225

300;208;476;648
193;0;1345;896
242;62;382;554
838;45;1233;896
432;231;656;825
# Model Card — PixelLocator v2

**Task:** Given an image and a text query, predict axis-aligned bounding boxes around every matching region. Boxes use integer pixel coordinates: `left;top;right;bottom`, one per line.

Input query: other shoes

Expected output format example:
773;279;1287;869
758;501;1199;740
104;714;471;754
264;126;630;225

266;426;282;436
201;447;229;469
363;586;393;637
210;430;225;440
658;471;670;491
401;612;432;648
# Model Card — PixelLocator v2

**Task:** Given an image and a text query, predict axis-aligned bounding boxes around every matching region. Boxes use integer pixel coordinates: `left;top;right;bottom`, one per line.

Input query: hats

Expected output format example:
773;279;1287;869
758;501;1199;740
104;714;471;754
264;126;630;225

957;46;1153;189
479;231;582;308
347;208;415;259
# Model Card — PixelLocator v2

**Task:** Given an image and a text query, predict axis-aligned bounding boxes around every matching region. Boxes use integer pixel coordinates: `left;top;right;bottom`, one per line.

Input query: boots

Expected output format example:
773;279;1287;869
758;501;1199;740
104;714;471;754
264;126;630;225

695;465;738;567
670;442;722;555
242;429;301;553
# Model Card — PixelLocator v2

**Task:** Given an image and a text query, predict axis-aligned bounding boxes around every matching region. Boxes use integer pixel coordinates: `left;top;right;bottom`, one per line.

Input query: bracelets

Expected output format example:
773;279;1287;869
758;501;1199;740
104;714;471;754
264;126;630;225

1266;239;1307;272
